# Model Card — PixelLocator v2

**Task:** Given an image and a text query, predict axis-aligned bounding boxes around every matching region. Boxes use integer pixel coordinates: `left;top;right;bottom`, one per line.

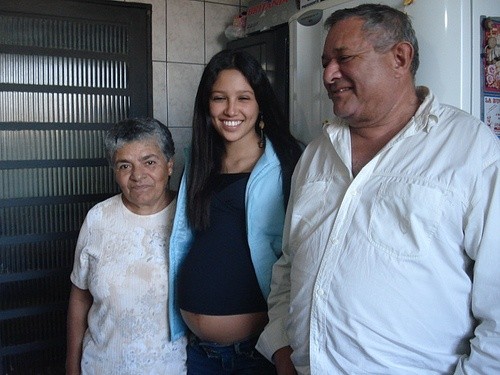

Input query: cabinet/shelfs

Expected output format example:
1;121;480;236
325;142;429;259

224;22;290;156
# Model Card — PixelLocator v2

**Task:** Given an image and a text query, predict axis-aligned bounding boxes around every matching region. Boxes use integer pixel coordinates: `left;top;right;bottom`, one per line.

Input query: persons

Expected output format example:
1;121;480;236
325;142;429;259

168;50;307;375
66;116;187;375
255;3;500;375
